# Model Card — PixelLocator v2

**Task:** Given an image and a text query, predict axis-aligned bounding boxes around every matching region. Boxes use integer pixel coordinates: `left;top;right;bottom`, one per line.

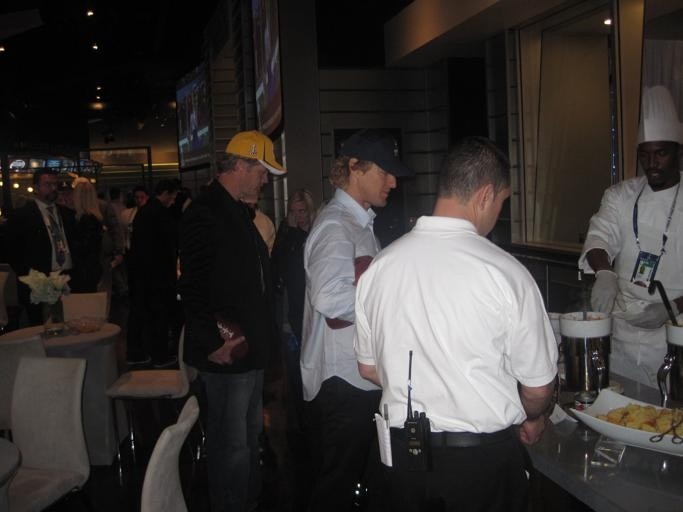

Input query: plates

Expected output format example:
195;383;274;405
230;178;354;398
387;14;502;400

569;388;683;457
65;316;105;333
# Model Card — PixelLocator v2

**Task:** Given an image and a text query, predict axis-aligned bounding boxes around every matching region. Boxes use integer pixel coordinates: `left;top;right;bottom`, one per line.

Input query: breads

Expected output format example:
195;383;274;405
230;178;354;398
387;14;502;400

598;404;683;435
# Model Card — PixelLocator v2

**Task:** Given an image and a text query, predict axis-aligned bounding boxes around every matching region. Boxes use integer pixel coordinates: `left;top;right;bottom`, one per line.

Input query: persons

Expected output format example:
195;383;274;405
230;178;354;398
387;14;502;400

300;126;415;512
348;132;559;511
179;131;290;512
5;168;97;326
576;120;682;394
72;176;316;367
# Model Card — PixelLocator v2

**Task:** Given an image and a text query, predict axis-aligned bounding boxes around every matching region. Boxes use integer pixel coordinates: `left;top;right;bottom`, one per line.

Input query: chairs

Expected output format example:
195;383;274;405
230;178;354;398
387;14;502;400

0;336;48;440
141;395;200;512
0;264;24;330
0;356;90;512
62;292;111;321
0;272;10;336
105;325;200;474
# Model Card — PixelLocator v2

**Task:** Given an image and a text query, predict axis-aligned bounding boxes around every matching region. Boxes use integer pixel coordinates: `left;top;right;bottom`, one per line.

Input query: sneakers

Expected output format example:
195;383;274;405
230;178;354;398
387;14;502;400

126;356;151;365
154;356;177;368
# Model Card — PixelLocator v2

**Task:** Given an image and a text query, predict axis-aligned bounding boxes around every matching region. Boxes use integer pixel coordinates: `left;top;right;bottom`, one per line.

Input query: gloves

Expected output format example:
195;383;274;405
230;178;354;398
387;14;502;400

590;269;626;314
625;300;680;329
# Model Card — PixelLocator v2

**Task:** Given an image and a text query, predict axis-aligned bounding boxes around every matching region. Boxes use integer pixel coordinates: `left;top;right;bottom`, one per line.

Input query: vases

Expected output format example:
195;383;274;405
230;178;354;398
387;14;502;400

43;302;60;322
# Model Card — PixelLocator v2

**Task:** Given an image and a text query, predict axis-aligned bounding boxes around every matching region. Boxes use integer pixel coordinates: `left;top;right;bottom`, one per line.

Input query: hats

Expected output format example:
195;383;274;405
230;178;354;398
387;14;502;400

225;130;287;176
341;126;411;177
637;85;683;146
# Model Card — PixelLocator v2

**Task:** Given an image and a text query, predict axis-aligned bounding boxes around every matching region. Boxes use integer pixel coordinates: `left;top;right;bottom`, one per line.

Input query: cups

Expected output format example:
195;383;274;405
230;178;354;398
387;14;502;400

559;310;613;403
658;314;683;408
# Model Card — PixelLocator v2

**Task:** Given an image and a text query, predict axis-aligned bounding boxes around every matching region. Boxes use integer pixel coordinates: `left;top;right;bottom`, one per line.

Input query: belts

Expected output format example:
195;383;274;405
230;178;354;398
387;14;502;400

426;424;514;449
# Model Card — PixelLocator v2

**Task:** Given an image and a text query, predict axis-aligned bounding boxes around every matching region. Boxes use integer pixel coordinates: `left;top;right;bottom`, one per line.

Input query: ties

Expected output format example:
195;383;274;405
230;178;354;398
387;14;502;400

45;207;65;266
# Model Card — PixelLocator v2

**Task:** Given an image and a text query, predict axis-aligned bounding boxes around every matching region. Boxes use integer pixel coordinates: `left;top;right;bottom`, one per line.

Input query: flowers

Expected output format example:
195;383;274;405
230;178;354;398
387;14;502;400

18;268;71;305
